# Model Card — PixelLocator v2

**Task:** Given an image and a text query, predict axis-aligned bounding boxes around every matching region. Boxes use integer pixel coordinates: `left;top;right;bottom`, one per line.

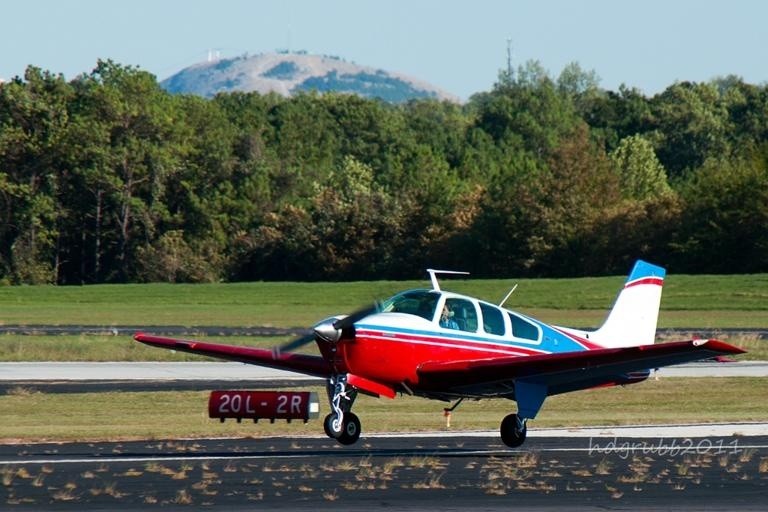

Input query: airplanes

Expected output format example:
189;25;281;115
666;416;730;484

131;257;750;448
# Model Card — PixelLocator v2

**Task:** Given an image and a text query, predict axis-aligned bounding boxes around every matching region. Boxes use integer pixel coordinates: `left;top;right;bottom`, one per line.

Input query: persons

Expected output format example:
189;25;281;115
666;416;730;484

439;304;459;330
